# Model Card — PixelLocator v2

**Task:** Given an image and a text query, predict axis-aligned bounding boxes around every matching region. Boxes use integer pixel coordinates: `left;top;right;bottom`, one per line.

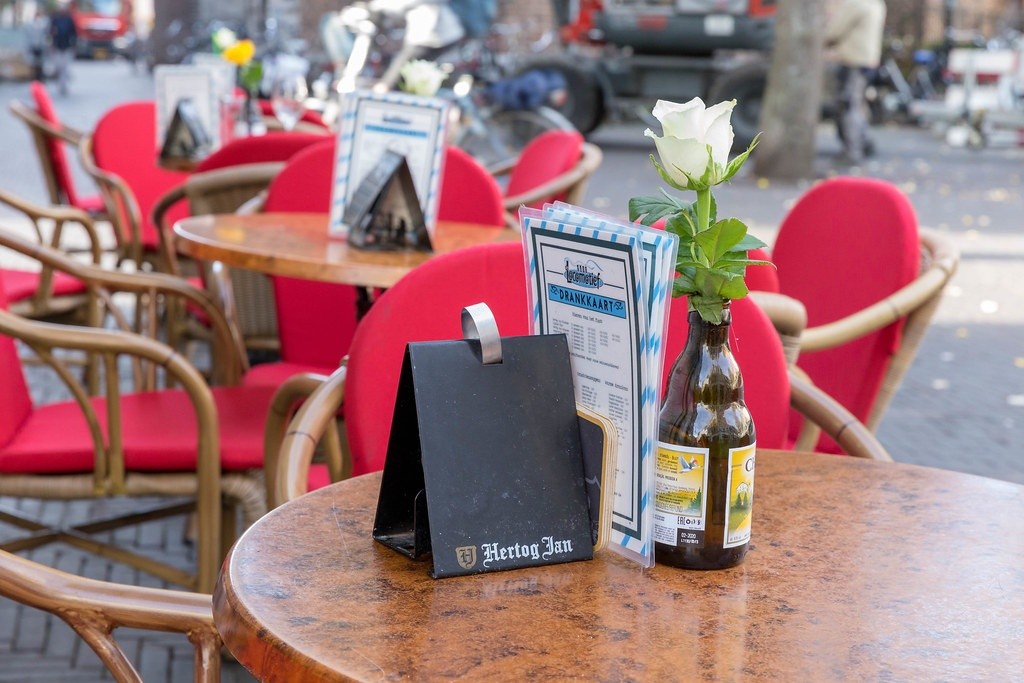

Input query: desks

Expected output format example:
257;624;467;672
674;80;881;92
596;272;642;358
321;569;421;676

174;212;526;322
216;445;1024;683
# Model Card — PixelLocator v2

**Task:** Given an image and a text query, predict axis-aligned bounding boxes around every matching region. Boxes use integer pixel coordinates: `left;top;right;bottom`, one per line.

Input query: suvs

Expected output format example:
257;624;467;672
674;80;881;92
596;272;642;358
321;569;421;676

507;1;771;155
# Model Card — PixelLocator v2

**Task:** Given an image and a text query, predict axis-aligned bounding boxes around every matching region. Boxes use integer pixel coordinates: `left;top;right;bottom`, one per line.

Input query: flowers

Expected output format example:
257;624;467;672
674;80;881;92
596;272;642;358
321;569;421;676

397;59;454;94
213;29;264;136
625;95;776;325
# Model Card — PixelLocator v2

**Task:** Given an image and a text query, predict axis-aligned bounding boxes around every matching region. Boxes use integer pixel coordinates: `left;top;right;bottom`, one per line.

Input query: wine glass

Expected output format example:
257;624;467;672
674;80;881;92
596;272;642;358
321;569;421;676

272;70;309;130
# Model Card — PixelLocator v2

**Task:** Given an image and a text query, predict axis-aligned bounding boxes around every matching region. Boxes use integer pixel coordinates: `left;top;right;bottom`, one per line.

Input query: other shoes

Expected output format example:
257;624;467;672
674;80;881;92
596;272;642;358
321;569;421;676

828;153;856;166
864;140;875;155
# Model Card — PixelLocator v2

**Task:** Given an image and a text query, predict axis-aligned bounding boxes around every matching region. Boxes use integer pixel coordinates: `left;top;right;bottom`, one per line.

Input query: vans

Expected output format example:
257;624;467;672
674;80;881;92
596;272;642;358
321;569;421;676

68;0;133;62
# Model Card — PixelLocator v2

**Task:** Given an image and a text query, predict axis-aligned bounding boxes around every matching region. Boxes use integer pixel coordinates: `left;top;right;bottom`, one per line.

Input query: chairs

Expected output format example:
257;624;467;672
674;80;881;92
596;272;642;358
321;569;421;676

0;81;957;683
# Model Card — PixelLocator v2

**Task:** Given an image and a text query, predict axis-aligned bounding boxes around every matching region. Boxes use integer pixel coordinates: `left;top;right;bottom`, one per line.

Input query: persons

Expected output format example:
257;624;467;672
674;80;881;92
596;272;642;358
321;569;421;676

336;0;465;93
490;0;554;53
822;0;887;169
50;6;77;95
